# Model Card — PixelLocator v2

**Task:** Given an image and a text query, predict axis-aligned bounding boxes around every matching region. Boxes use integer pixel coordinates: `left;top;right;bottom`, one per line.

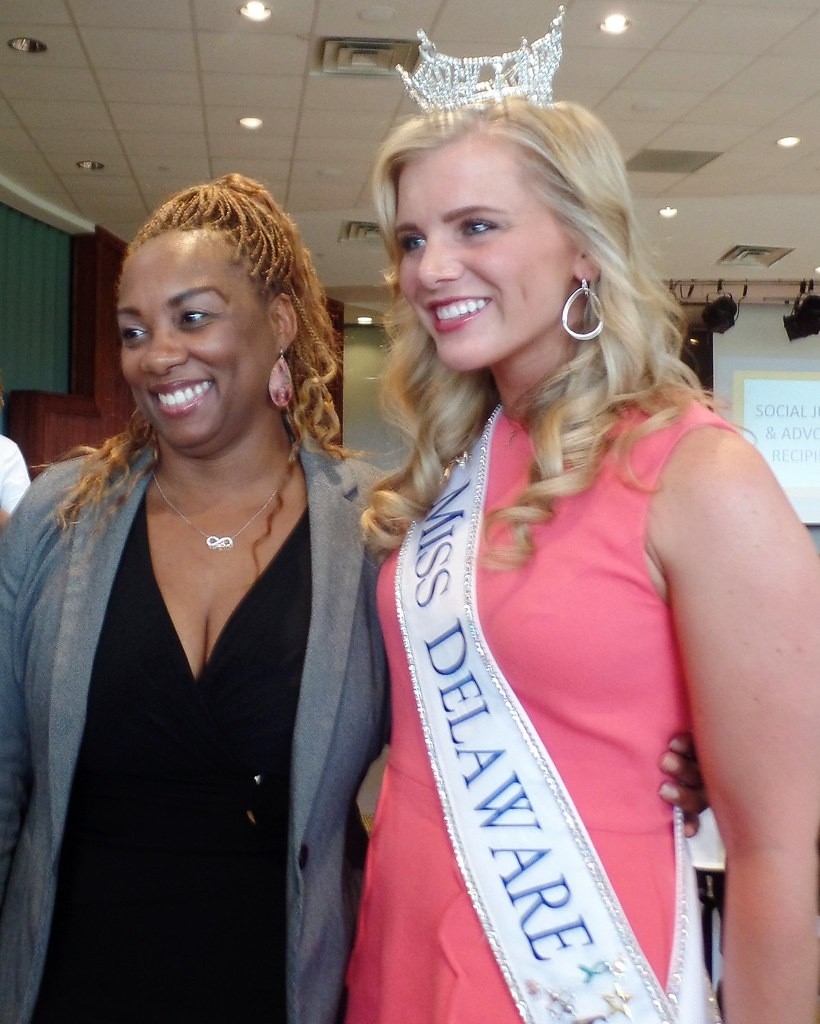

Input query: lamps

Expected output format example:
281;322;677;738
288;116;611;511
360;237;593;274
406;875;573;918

702;278;748;335
783;278;820;342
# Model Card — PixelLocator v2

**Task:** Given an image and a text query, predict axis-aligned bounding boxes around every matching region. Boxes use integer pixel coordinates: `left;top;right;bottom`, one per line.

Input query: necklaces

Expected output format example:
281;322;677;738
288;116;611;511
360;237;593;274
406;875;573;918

150;467;285;552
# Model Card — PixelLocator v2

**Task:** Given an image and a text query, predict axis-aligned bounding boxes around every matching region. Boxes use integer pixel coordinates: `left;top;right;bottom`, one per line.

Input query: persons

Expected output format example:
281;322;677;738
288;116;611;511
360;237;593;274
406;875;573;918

345;80;820;1023
0;431;33;530
0;171;712;1024
